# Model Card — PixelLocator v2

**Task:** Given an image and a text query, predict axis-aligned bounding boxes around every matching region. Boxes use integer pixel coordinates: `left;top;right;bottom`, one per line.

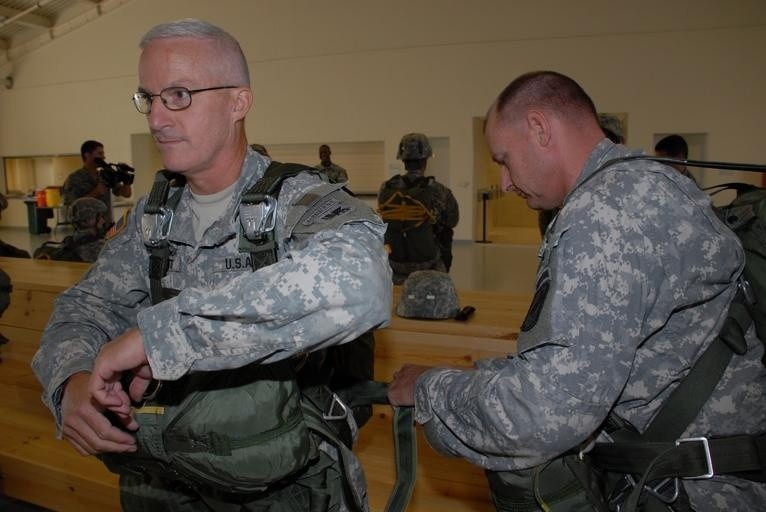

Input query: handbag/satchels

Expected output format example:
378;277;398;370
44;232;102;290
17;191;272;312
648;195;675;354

484;449;615;512
94;356;314;496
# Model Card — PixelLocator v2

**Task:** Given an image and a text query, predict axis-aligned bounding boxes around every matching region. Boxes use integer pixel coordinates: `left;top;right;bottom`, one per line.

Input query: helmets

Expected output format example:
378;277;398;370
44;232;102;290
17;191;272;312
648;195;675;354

394;269;462;320
68;196;108;231
395;133;433;161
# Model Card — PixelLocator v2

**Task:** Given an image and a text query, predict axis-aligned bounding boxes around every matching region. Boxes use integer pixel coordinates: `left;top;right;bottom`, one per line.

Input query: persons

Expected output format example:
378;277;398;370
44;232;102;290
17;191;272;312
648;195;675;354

30;18;393;512
33;197;109;263
313;144;348;193
388;71;766;512
376;134;460;286
63;140;131;231
655;134;701;191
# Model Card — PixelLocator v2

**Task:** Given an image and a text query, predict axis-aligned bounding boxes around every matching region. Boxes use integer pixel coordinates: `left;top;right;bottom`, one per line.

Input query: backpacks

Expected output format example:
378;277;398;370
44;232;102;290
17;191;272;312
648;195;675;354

553;155;765;482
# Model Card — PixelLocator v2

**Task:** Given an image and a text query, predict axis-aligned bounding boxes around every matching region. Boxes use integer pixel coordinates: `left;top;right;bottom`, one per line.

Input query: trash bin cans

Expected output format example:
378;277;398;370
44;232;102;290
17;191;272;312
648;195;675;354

24;201;51;235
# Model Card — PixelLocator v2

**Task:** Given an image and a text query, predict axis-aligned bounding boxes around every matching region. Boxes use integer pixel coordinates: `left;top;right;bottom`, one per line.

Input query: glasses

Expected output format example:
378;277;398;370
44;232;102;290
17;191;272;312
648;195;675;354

131;85;238;115
